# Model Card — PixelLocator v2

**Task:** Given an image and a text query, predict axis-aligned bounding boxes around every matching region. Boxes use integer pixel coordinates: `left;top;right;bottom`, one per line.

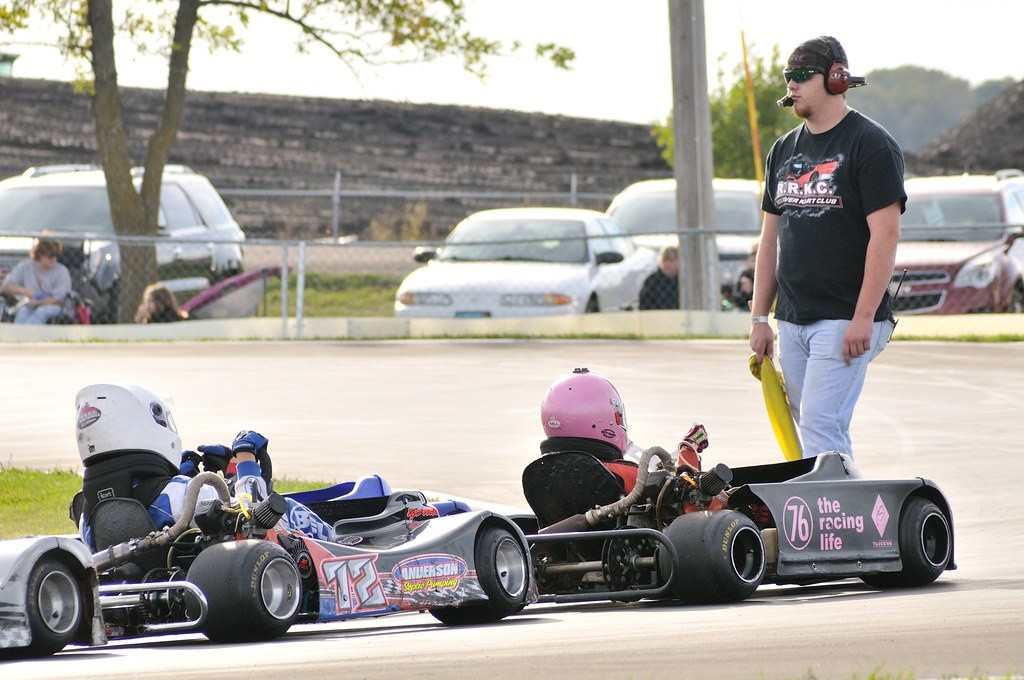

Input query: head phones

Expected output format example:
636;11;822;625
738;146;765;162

821;36;849;95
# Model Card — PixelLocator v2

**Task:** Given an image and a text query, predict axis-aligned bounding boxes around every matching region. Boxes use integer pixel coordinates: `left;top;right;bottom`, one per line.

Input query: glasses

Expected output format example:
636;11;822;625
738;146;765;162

782;68;823;83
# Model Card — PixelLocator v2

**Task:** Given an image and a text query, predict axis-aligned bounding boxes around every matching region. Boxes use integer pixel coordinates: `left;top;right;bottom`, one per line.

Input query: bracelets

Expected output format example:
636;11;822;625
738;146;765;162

751;315;768;323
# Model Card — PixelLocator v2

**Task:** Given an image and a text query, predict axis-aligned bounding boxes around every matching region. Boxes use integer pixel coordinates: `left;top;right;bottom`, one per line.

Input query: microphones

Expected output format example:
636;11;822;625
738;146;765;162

776;96;793;109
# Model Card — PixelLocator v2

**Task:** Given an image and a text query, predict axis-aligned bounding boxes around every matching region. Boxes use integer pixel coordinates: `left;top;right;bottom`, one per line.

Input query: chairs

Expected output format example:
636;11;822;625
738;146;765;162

86;496;158;549
513;449;625;519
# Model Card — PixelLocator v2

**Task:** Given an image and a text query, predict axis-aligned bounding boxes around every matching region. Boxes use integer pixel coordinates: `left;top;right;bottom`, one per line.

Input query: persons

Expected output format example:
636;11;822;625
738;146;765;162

732;251;756;298
638;244;679;310
135;282;182;323
750;32;912;479
69;380;335;542
2;230;74;325
737;267;755;301
541;368;730;514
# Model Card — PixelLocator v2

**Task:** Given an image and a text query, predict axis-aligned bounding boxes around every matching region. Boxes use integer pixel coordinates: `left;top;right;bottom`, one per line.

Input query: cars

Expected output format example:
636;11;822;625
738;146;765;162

613;174;781;307
397;208;655;311
0;159;244;325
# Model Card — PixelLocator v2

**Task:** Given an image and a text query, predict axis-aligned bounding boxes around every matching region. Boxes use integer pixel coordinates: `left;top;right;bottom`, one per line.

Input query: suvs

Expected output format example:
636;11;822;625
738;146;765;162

889;166;1022;313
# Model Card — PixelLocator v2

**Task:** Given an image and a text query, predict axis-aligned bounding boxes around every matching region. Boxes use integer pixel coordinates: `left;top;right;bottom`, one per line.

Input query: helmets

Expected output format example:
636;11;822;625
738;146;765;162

76;381;181;474
541;368;628;459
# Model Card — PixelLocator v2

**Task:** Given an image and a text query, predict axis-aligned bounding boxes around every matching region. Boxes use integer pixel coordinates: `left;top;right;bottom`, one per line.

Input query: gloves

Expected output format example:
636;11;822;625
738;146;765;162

681;423;709;454
231;429;268;462
181;451;202;477
